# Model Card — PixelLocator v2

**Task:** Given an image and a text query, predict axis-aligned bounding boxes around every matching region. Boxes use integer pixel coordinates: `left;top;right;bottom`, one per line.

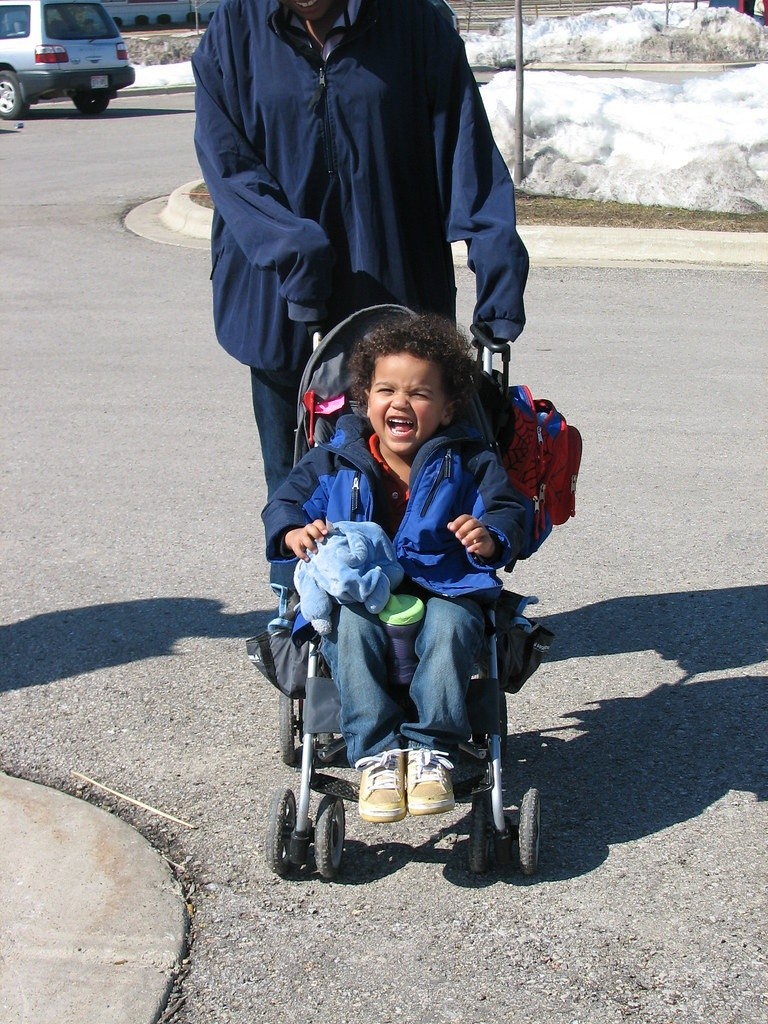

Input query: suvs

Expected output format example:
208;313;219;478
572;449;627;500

0;1;136;120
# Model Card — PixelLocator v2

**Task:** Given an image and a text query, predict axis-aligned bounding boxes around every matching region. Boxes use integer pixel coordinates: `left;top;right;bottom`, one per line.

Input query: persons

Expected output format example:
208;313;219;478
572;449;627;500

262;315;530;821
193;0;536;624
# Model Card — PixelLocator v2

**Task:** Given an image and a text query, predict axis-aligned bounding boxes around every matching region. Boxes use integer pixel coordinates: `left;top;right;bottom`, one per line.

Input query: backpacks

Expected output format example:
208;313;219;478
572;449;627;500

503;383;584;574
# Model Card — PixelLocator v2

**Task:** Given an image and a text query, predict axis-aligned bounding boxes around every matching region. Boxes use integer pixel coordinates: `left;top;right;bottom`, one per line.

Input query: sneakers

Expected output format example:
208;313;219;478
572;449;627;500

352;748;406;824
404;745;457;817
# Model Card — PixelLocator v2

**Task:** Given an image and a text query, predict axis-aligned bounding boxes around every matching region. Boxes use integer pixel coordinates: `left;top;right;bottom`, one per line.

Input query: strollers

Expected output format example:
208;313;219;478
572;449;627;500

264;301;543;883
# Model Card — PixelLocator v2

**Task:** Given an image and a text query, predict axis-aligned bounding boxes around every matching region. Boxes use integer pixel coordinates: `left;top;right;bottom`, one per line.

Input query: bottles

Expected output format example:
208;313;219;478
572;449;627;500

379;596;425;684
